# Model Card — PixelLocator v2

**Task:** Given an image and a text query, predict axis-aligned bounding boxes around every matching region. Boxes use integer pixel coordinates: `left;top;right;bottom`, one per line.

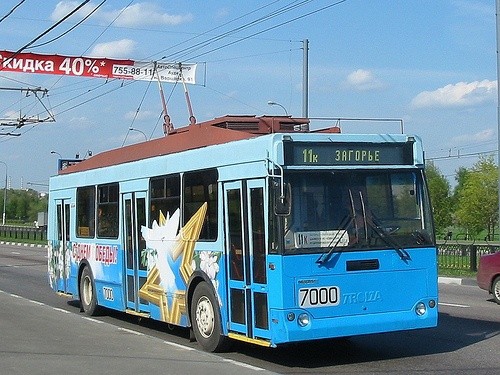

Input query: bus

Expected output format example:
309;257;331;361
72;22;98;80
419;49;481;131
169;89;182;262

47;116;439;353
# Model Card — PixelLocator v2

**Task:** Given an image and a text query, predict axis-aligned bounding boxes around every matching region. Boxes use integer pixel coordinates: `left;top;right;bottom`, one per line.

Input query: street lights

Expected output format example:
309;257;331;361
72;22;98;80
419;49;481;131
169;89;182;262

129;128;147;141
268;101;288;114
51;151;62;159
